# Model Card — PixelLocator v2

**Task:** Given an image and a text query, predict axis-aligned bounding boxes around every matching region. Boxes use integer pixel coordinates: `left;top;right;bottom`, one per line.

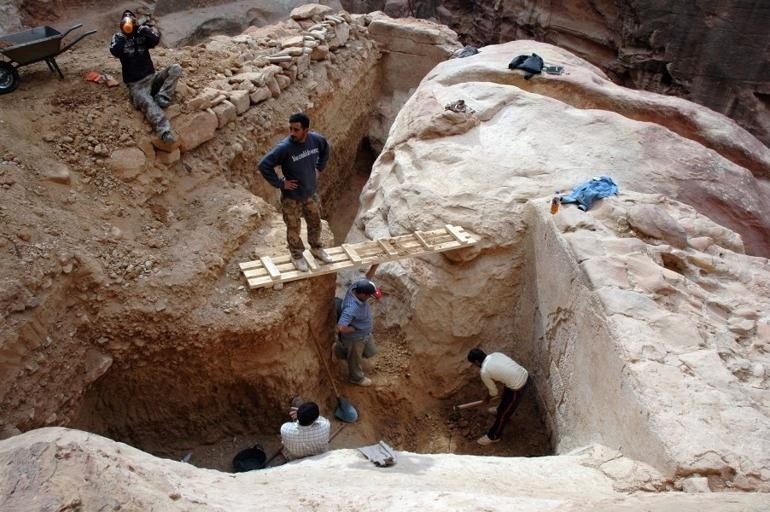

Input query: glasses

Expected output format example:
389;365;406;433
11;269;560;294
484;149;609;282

124;13;132;16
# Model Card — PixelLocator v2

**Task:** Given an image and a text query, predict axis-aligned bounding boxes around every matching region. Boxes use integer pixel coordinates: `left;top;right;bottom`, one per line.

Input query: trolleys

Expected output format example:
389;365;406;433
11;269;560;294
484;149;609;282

0;21;99;95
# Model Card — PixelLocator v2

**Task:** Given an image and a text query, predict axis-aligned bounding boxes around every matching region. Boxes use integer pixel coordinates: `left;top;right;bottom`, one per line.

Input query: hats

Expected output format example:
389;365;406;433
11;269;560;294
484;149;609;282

356;280;382;299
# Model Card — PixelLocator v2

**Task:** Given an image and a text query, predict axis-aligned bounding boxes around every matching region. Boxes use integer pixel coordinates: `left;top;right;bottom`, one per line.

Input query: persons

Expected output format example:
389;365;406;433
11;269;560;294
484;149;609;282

281;402;330;461
256;113;334;272
331;278;379;387
110;10;183;146
468;348;532;445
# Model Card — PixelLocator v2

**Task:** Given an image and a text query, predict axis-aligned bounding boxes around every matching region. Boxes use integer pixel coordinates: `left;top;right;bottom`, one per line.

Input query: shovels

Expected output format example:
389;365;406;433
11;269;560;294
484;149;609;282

307;320;358;423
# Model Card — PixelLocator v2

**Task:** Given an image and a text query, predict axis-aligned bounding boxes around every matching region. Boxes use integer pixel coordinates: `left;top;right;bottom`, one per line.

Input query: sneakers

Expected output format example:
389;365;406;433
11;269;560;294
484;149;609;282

162;132;175;146
489;407;497;413
291;257;307;272
309;248;332;264
155;94;168;108
349;377;372;386
331;342;338;365
476;434;500;445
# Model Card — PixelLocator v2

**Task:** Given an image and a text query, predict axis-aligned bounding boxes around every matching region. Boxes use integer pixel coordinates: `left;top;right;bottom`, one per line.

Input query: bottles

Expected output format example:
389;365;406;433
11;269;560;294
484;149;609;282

550;192;560;215
122;13;133;34
